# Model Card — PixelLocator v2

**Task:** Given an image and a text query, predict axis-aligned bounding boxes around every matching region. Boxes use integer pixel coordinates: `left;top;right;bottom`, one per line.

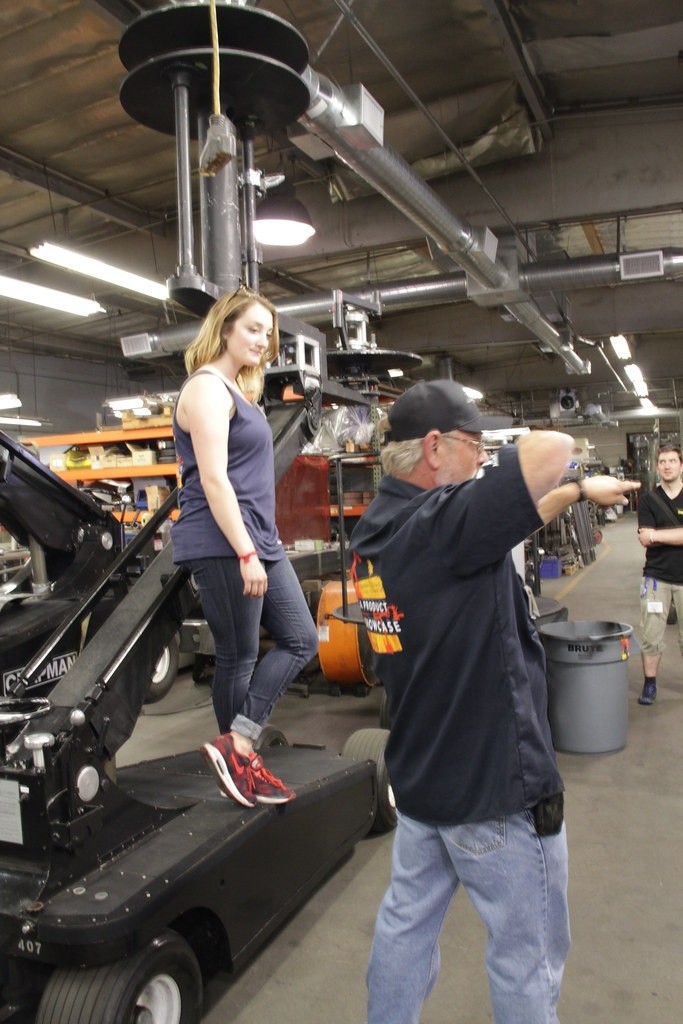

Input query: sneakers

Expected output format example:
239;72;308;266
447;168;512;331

638;684;657;704
219;753;296;804
202;734;258;808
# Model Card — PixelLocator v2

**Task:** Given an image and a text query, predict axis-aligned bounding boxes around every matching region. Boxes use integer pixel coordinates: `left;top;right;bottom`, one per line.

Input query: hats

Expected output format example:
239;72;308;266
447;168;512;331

384;379;513;446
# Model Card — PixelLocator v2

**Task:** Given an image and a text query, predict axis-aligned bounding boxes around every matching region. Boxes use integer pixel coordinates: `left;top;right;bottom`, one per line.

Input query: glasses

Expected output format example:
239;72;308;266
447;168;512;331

442;435;485;453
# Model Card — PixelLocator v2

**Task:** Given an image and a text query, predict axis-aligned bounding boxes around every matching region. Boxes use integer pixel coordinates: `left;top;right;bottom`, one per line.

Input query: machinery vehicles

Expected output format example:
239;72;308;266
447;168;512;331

0;0;606;1024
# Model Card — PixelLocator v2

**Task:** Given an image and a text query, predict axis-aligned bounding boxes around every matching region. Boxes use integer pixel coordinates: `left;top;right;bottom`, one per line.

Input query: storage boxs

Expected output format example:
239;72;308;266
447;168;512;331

540;558;563;578
49;442;158;472
137;484;170;511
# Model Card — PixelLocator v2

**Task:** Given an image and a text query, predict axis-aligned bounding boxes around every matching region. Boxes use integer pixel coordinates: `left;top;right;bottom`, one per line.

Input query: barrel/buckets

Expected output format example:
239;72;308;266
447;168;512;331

535;620;637;754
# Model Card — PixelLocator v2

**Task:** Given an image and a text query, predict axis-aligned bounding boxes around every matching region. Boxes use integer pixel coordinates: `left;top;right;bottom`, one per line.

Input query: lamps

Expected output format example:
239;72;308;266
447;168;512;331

0;166;170;316
609;288;652;408
0;321;51;426
253;149;316;248
103;319;157;419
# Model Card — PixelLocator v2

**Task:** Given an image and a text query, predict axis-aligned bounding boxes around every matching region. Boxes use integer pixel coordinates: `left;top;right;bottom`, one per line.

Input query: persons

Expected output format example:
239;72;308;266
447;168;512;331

171;286;320;808
348;378;641;1024
638;445;683;705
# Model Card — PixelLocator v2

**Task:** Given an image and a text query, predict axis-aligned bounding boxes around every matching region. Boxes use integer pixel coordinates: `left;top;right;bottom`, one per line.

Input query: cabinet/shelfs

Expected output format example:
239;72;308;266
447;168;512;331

23;426;182;523
331;405;382;519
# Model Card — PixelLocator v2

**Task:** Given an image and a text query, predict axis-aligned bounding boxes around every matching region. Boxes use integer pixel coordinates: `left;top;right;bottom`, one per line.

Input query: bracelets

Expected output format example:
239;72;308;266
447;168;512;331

572;479;587;502
238;551;258;564
